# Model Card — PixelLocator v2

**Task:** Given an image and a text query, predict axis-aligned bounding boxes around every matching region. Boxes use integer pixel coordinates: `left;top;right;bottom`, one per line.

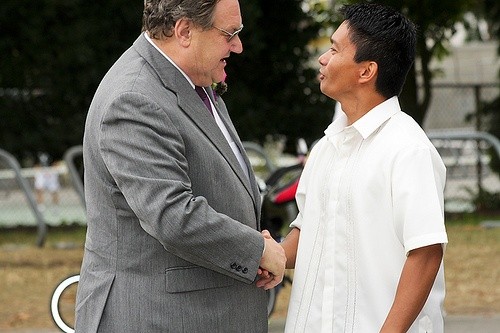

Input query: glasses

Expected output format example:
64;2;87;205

194;19;244;41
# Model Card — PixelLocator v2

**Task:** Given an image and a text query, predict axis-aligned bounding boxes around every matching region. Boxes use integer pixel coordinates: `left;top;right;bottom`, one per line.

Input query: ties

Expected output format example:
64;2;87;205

195;85;214;118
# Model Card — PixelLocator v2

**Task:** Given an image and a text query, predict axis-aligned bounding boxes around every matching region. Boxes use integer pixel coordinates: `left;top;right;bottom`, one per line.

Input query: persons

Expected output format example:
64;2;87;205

31;129;68;211
257;2;449;333
73;0;288;333
